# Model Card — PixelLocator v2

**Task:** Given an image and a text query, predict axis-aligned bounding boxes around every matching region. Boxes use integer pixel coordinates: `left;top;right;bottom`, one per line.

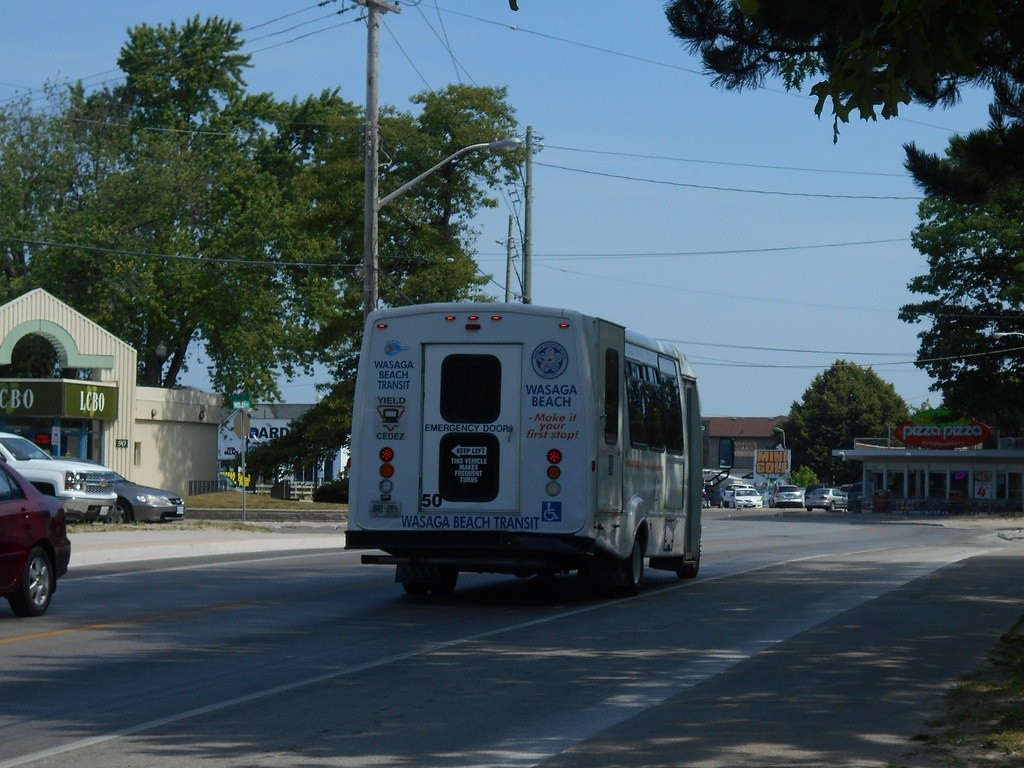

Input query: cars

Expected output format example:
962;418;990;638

702;485;724;508
768;485;803;508
52;456;186;524
840;484;853;491
728;488;763;508
0;460;72;617
723;484;754;506
804;488;848;512
847;483;863;511
0;432;118;523
220;475;243;492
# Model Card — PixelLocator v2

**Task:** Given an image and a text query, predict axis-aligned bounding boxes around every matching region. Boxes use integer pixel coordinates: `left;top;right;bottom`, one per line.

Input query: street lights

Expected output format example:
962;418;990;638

364;138;522;319
774;427;786;468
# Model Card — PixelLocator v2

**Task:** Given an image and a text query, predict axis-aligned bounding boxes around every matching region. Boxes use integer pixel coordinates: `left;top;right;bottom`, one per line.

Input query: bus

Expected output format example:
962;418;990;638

344;302;703;603
